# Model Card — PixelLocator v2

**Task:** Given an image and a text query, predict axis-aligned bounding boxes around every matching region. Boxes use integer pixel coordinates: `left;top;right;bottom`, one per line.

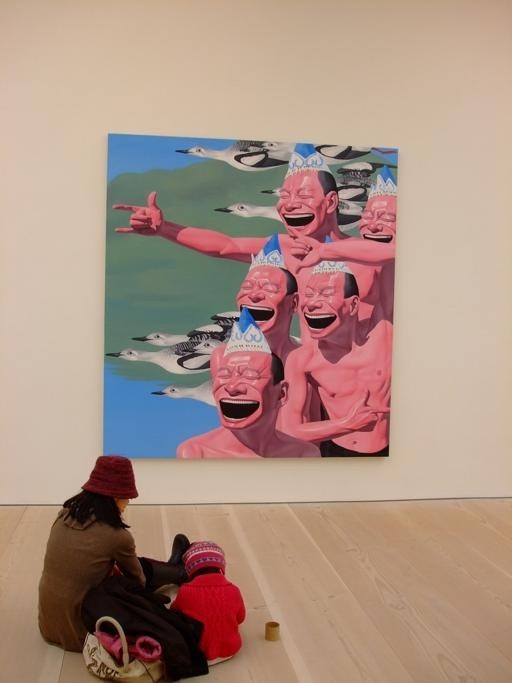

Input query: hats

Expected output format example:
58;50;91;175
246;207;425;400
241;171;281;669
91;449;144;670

182;540;226;578
370;167;398;197
314;234;355;274
287;144;335;174
81;455;138;499
219;305;274;354
249;230;290;268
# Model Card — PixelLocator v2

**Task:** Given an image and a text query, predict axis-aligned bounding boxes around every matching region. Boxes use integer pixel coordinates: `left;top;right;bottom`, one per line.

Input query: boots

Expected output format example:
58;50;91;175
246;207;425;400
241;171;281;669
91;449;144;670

136;533;190;593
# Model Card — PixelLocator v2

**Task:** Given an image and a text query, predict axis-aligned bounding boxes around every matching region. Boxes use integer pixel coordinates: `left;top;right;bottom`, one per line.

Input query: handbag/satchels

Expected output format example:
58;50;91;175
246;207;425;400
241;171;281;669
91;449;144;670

81;616;164;683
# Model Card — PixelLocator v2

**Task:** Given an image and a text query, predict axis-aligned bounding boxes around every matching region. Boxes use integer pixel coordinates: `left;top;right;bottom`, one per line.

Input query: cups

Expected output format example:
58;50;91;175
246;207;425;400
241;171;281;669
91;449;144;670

264;621;281;641
